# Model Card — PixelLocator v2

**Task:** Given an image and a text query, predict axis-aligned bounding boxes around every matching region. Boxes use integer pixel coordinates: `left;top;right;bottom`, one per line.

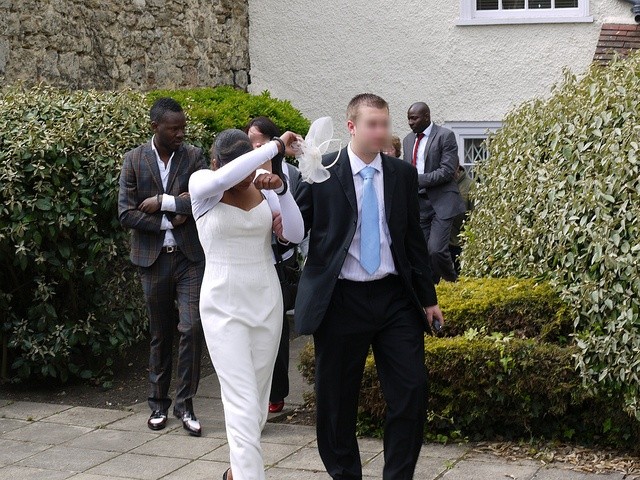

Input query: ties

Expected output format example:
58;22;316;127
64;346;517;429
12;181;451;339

412;133;424;168
359;167;380;275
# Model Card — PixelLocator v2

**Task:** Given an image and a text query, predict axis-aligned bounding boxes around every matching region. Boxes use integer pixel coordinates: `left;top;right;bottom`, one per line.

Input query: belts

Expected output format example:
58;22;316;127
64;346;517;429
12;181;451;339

161;245;180;253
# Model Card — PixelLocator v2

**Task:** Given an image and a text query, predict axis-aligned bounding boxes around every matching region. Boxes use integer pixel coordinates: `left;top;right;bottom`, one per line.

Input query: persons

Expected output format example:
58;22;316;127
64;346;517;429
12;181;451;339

271;92;445;480
383;135;401;158
448;157;478;275
187;129;306;480
245;115;303;417
117;98;205;437
402;101;466;285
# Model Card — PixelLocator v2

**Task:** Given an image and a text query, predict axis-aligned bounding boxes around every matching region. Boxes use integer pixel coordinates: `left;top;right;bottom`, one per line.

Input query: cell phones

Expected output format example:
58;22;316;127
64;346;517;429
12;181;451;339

430;318;445;339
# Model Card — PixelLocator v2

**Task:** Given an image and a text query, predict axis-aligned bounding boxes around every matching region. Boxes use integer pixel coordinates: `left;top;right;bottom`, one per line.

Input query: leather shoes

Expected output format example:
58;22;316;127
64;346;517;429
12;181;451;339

269;401;284;413
173;408;201;435
148;409;168;429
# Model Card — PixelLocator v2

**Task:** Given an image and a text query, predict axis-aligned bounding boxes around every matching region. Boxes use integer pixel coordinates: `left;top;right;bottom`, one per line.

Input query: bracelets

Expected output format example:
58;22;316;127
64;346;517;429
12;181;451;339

276;180;287;197
272;135;285;154
156;194;160;206
